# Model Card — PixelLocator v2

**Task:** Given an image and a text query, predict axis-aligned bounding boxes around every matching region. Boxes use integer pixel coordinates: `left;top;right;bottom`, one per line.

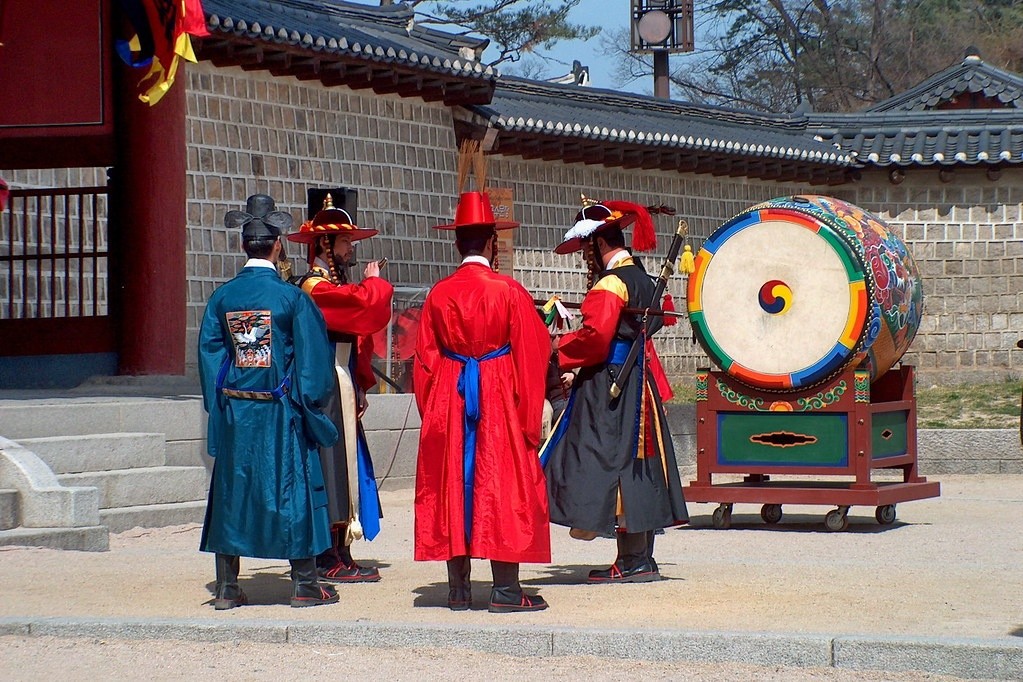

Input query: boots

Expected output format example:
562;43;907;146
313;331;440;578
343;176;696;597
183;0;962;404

588;528;660;582
215;553;248;609
336;523;379;582
316;529;360;583
488;559;547;612
448;556;472;611
290;557;340;608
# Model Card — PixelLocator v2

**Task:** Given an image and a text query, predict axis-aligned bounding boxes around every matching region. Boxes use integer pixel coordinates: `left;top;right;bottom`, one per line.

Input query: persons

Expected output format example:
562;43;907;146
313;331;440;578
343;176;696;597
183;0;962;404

536;196;689;582
289;198;393;584
197;195;339;608
535;308;576;453
413;191;550;613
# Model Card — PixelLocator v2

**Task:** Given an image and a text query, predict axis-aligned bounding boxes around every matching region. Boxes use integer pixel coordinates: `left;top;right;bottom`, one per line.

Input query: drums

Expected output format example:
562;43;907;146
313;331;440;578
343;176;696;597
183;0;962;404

689;194;925;396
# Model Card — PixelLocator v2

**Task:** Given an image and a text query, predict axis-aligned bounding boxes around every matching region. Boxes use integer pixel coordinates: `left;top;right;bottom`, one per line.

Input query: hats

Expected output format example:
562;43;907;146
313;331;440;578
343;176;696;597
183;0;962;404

432;139;520;230
224;194;292;239
285;186;378;287
552;193;677;255
